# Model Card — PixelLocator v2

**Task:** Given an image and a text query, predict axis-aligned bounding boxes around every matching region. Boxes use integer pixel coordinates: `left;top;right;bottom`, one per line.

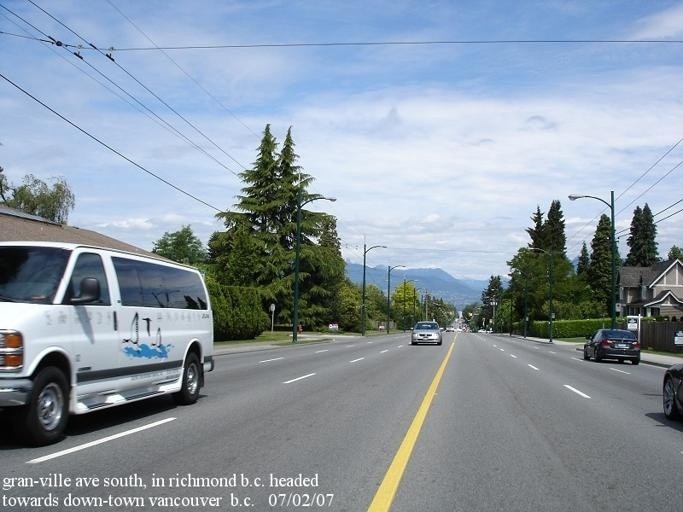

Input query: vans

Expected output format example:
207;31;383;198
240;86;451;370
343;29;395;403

0;240;214;446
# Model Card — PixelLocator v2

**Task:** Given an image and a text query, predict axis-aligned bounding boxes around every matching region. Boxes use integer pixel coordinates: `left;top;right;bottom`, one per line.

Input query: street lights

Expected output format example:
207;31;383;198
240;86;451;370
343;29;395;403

362;244;425;336
569;191;616;329
487;247;552;342
293;197;336;341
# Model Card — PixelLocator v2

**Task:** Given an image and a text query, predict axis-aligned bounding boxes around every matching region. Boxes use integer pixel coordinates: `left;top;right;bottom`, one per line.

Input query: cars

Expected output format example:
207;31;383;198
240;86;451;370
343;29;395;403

584;329;640;365
410;320;443;345
663;363;683;420
